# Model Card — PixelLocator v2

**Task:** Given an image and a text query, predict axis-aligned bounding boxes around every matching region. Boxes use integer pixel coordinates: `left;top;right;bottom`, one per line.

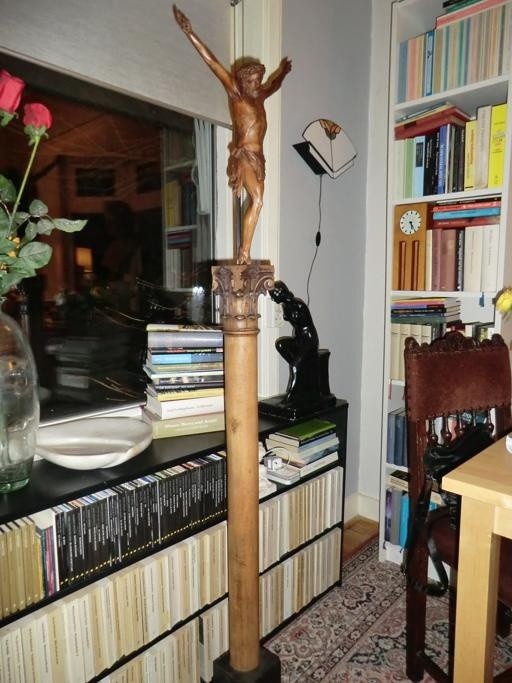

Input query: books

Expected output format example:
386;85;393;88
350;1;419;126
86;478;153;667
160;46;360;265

143;319;226;439
257;415;344;643
164;131;208;291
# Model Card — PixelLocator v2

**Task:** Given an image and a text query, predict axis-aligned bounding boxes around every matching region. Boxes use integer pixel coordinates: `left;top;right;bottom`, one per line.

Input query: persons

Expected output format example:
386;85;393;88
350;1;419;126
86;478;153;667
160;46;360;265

170;5;295;264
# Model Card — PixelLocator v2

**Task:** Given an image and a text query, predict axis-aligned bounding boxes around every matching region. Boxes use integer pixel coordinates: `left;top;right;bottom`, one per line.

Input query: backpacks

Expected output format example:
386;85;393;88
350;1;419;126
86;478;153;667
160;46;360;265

420;420;496;532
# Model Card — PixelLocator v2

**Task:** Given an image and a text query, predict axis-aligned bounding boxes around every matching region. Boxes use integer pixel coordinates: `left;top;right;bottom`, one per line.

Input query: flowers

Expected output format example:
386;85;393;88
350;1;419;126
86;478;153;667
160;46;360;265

0;68;60;303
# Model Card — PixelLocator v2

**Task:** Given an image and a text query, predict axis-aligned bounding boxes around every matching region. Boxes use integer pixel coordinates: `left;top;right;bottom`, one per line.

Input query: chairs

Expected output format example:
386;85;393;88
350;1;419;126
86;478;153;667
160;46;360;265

401;328;511;680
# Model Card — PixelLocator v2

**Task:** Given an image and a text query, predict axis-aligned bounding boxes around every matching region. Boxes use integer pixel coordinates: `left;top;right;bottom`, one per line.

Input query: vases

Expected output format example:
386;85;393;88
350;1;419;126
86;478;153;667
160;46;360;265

0;299;44;492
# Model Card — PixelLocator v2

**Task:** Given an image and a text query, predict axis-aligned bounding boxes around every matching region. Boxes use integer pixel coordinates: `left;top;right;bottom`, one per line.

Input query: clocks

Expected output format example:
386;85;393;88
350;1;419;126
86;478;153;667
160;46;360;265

399;206;425;237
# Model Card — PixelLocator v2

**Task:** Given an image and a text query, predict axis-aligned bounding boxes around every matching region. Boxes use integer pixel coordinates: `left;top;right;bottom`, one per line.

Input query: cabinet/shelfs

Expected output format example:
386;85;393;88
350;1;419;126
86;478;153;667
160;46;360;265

1;397;351;677
376;0;510;572
158;127;205;315
